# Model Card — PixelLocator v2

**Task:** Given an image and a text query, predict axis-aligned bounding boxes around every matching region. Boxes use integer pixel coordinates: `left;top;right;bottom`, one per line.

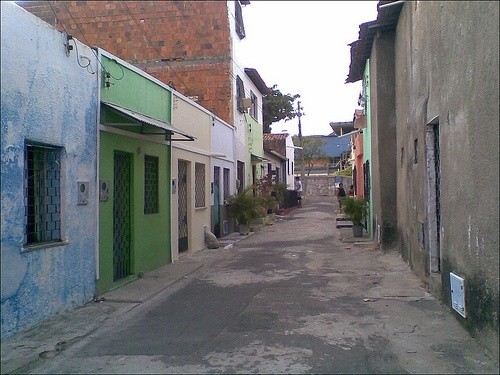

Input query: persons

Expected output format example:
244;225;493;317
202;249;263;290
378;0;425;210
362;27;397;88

335;182;346;213
294;176;302;209
348;185;355;198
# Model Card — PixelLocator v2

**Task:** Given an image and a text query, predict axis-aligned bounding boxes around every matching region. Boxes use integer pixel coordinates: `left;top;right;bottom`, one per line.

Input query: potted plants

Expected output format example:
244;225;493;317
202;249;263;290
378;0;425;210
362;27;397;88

226;185;272;234
254;194;276;215
337;196;369;238
